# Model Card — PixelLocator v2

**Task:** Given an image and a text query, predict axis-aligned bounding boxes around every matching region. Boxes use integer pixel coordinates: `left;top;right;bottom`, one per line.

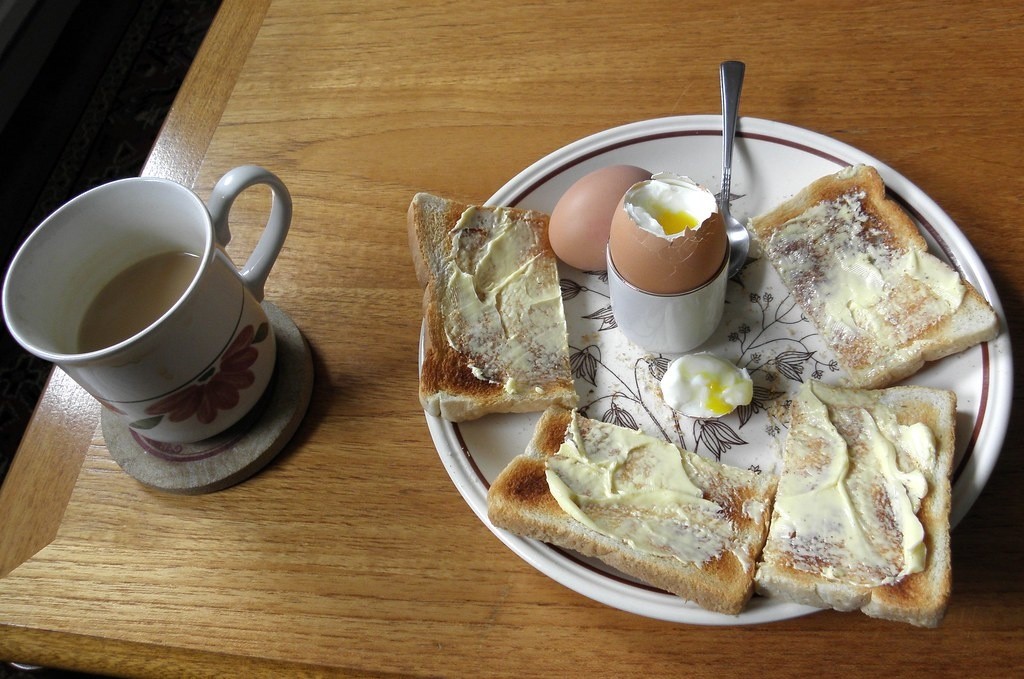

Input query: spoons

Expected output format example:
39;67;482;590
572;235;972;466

695;61;749;280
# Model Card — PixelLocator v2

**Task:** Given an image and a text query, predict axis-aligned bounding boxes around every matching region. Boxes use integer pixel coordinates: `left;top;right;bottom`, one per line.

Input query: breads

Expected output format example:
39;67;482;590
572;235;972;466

406;192;578;423
752;377;958;628
747;162;998;389
485;402;781;615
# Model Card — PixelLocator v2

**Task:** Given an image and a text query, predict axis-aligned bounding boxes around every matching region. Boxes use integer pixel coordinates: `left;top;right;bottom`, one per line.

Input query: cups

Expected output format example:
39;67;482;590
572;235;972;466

1;161;292;445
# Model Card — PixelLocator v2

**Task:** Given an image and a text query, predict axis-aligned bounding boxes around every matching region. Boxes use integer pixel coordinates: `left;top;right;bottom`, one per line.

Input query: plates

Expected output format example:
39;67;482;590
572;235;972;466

417;115;1013;625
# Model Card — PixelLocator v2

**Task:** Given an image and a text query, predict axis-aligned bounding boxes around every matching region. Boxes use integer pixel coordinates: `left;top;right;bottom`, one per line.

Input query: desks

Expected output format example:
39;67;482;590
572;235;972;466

0;0;1024;679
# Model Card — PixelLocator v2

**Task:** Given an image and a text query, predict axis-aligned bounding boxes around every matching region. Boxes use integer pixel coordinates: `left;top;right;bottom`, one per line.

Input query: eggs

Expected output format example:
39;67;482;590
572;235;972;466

610;170;726;295
548;163;658;272
659;351;755;419
